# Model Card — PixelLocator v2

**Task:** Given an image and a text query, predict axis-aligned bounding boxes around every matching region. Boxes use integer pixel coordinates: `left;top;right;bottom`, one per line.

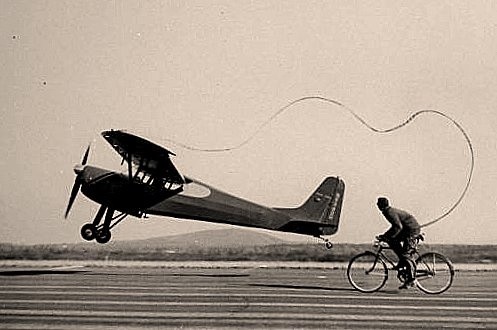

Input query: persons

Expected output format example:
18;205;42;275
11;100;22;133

376;197;420;289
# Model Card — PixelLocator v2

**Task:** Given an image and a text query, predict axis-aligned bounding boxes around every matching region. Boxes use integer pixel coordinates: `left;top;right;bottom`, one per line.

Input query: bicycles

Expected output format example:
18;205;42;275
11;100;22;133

346;234;454;294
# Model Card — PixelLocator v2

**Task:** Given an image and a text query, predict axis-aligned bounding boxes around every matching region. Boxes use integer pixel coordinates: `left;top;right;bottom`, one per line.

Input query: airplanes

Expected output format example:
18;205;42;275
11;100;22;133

65;129;345;249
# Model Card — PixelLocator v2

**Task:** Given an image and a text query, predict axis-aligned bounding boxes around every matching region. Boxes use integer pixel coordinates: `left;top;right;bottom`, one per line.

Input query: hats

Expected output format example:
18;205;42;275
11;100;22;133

376;198;389;207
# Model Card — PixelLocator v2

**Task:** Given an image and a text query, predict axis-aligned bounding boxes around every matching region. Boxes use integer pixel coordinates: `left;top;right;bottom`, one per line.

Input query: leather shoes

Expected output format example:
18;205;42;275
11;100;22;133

392;263;406;270
398;279;416;289
